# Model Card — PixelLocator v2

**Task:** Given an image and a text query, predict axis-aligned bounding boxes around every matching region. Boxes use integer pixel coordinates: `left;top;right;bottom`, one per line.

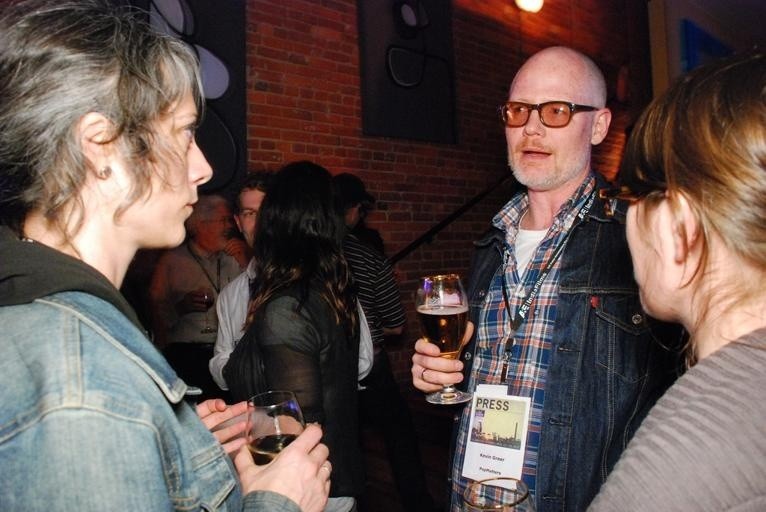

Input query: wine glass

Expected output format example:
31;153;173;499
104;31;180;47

195;285;220;334
413;274;477;404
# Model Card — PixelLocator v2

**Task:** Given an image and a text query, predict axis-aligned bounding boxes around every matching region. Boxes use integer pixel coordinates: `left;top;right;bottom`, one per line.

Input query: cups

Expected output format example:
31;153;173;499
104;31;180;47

245;389;305;465
464;476;536;512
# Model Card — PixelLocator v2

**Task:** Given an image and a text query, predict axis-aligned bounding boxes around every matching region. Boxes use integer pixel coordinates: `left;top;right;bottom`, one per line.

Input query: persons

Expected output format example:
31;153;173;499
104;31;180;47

0;0;333;512
411;46;697;512
586;48;766;512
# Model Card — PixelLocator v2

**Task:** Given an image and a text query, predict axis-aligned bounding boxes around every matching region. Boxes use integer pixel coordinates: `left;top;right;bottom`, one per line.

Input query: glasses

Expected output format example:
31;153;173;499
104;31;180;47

497;98;599;128
